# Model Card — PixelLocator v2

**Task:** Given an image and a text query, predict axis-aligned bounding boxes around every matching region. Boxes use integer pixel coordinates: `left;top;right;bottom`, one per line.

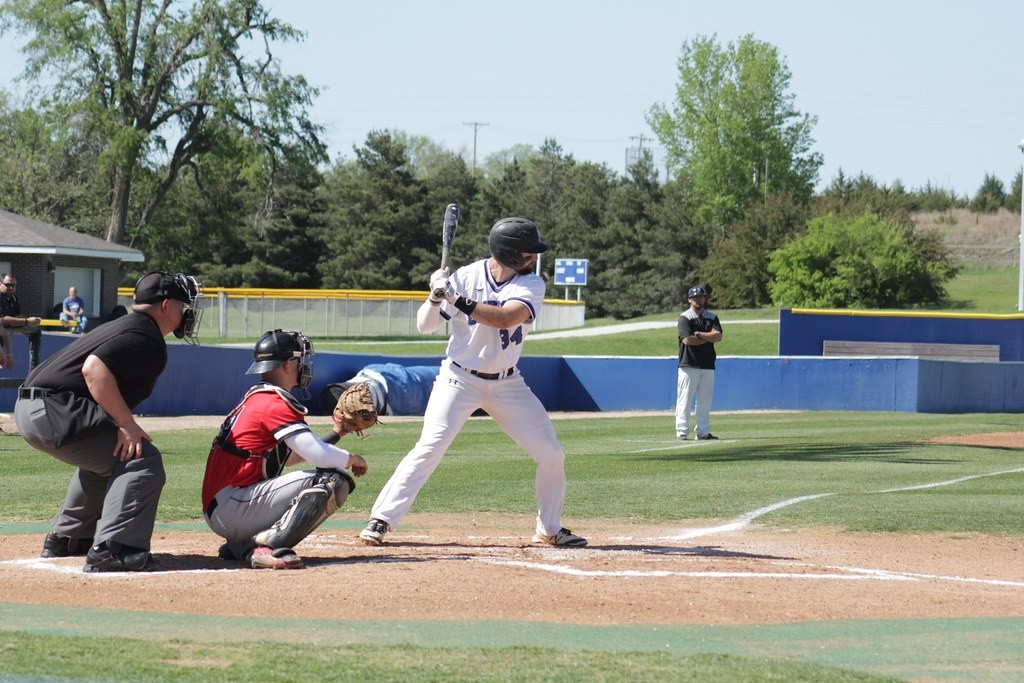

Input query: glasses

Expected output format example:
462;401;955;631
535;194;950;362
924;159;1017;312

1;282;16;288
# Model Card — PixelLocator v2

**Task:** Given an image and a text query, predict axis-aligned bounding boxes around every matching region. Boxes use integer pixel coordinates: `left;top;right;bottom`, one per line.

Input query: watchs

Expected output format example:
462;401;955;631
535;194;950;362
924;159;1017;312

695;331;699;337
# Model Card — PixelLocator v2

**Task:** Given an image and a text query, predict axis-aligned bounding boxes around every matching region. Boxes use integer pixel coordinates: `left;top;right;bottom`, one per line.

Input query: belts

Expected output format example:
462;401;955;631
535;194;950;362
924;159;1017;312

19;389;55;399
452;361;514;380
206;499;218;519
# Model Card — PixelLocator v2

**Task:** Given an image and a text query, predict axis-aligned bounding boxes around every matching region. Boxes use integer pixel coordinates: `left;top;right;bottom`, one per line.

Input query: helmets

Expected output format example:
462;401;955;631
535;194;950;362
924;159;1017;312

245;331;299;374
489;218;548;275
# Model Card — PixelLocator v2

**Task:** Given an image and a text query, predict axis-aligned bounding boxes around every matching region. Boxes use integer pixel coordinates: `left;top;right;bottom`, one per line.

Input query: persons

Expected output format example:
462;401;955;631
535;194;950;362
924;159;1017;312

14;270;203;572
676;285;723;441
0;273;41;433
203;329;369;570
59;287;87;335
360;217;588;547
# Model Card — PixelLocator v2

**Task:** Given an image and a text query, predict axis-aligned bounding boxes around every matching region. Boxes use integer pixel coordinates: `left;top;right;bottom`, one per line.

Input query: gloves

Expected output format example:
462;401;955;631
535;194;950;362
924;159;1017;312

430;278;460;306
428;266;452;302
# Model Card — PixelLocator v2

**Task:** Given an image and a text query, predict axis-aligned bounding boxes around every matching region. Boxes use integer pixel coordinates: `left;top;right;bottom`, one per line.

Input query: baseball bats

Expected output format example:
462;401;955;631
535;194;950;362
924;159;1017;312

434;203;461;297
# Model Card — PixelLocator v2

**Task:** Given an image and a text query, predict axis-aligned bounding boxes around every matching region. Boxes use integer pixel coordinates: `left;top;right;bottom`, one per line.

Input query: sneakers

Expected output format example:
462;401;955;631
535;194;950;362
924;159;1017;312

44;533;94;557
359;519;388;545
532;527;587;548
246;546;304;570
86;539;160;572
219;544;252;561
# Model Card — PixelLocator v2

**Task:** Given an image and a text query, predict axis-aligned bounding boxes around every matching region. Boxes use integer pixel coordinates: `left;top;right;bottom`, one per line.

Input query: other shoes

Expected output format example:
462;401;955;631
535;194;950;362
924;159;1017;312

679;435;687;439
695;433;718;440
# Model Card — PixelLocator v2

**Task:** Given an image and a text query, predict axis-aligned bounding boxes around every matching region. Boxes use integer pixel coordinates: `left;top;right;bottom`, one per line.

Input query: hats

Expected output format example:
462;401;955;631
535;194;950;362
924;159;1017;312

133;271;197;304
688;287;707;298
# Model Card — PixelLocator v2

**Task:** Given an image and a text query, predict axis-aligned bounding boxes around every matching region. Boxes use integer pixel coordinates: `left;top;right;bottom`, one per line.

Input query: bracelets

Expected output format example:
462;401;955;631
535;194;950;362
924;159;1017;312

6;354;13;357
24;317;27;326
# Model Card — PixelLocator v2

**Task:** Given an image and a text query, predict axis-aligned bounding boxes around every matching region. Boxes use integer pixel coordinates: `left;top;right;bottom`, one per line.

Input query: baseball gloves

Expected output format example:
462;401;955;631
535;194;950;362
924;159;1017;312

332;383;378;433
